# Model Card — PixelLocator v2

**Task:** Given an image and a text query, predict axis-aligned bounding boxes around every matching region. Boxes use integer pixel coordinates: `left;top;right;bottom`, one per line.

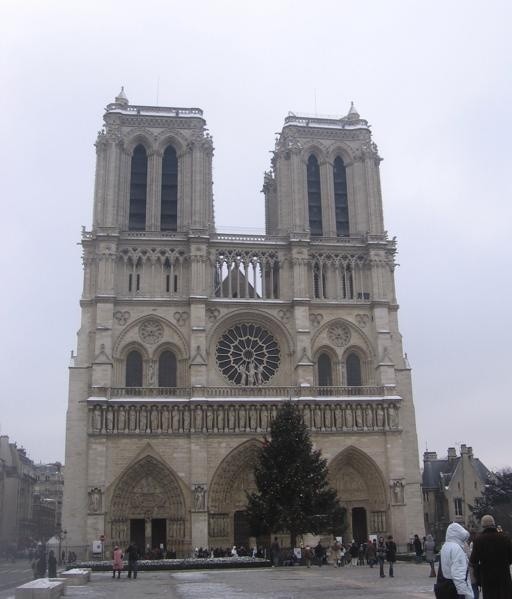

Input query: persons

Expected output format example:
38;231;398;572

264;536;397;577
112;544;138;579
414;516;511;599
195;544;264;558
62;550;76;564
31;550;57;578
138;541;176;560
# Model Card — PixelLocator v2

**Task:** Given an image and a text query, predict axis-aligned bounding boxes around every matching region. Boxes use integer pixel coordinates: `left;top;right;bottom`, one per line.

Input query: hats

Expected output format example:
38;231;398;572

480;514;497;527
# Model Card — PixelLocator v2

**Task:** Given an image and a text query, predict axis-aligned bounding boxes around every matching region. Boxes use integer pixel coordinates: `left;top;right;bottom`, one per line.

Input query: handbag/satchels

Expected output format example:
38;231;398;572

433;551;471;599
